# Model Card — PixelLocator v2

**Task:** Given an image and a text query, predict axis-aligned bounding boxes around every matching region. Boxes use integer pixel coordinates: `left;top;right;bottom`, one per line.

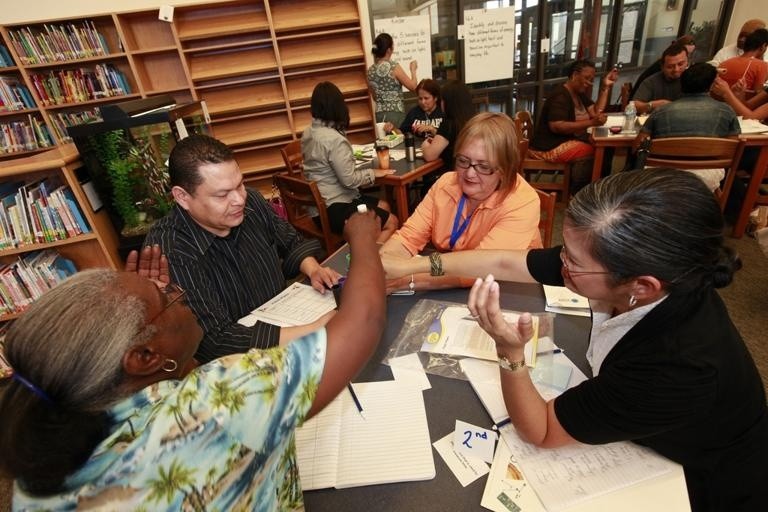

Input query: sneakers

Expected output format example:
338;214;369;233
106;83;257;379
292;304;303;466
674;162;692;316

748;206;768;236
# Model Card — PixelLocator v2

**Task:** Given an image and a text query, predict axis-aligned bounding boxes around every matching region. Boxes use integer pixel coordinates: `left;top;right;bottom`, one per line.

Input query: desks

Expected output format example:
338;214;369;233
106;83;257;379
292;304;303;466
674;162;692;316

294;242;590;511
352;139;445;228
589;110;768;241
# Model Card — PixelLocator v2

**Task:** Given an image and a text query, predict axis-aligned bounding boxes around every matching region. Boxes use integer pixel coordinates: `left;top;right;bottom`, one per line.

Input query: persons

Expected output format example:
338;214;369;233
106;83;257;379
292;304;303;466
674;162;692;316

528;58;618;179
630;20;768;161
366;32;418;129
378;113;543;293
141;134;346;366
384;79;478;168
296;82;390;239
379;167;767;511
0;204;388;512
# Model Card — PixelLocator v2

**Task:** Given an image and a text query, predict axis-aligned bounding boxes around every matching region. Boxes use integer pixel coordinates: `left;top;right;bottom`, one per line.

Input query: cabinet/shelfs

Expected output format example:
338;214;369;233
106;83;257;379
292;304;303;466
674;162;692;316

168;0;379;203
1;143;130;375
0;5;203;161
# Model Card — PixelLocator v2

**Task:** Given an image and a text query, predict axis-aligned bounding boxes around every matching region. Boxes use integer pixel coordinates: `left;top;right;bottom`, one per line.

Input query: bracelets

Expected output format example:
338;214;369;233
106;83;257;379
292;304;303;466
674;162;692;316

408;274;415;293
430;250;443;276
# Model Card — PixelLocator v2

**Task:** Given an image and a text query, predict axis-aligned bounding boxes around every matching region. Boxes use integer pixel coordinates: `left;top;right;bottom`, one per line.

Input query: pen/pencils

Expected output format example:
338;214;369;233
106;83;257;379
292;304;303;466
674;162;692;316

492;418;511;431
348;383;366;419
742;56;754;80
536;349;564;357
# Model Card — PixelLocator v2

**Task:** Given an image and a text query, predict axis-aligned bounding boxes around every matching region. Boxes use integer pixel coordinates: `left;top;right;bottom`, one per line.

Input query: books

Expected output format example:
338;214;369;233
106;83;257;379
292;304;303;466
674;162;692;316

0;20;132;154
294;380;437;492
0;319;16;370
0;177;92;251
0;248;78;315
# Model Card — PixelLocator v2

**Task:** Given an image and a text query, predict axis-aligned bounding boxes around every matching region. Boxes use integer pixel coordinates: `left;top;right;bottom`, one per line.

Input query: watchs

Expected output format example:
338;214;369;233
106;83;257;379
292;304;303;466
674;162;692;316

498;356;524;372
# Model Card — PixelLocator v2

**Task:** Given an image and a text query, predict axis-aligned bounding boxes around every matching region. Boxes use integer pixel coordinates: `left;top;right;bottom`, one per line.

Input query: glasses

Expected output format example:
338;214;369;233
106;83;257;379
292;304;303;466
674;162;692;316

136;282;188;334
455;153;499;175
560;238;613;273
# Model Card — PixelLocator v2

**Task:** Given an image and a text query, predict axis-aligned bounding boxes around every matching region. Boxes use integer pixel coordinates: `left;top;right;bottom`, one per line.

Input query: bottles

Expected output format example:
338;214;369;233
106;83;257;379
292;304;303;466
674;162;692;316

404;126;416;161
625;102;636;130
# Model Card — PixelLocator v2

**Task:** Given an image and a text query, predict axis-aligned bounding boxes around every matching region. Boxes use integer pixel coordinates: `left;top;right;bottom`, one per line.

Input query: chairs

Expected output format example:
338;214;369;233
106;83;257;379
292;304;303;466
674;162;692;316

274;173;346;259
639;136;746;222
534;188;558;249
512;108;573;213
281;141;304;178
469;63;566;114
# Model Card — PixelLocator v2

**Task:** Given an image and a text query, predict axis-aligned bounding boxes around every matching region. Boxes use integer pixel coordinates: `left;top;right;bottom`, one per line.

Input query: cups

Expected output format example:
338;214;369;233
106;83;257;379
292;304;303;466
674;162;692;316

377;146;390;169
375;122;386;139
